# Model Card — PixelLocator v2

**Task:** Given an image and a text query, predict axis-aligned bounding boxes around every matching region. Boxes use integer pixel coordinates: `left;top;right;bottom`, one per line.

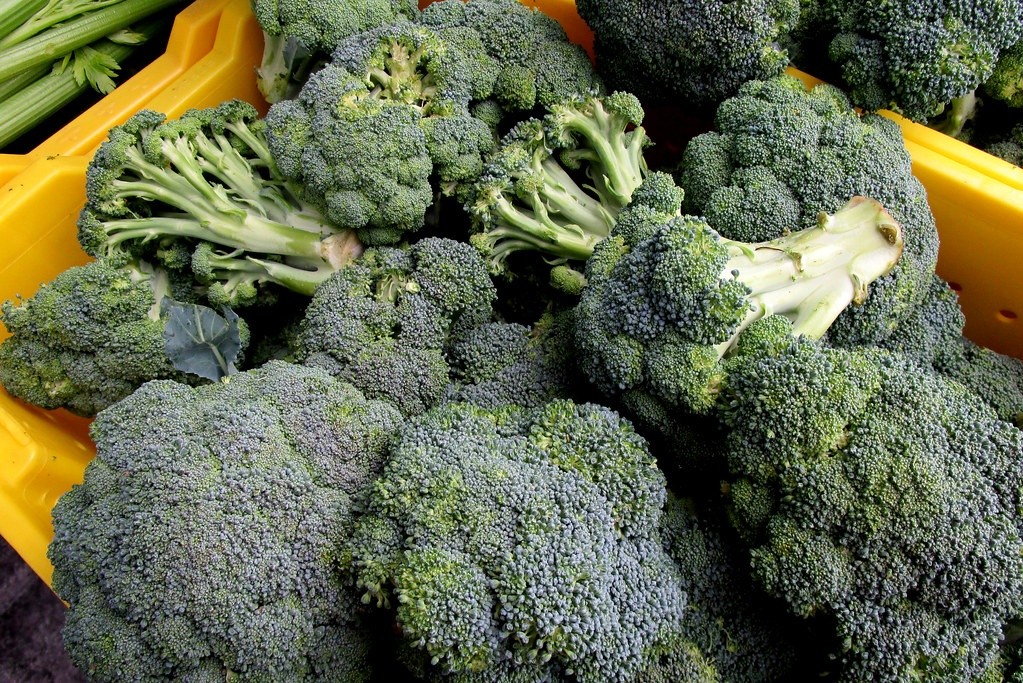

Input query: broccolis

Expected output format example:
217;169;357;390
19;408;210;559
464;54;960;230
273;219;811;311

0;0;1023;683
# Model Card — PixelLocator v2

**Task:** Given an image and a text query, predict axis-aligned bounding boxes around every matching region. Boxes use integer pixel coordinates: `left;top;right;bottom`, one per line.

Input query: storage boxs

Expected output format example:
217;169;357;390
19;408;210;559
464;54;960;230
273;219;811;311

0;0;1023;683
